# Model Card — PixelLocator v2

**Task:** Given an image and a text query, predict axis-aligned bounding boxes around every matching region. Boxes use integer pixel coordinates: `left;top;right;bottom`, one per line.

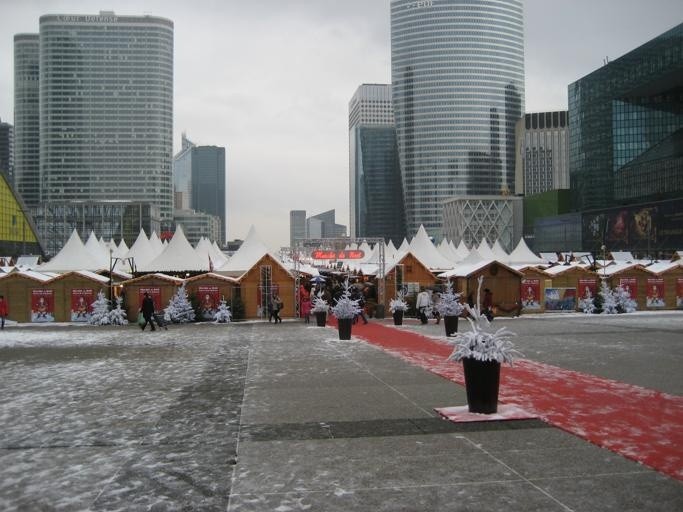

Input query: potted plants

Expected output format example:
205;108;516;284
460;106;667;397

443;317;525;414
309;272;468;341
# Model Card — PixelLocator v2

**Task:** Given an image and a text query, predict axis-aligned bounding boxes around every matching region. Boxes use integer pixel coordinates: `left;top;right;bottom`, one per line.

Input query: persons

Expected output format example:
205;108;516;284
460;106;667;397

522;286;539;309
431;287;443;323
646;283;665;307
578;286;594;309
351;291;368;325
138;291;156;332
301;296;312;323
0;295;7;329
268;299;274;323
415;286;430;323
482;288;493;317
624;284;637;306
464;290;477;320
271;294;282;324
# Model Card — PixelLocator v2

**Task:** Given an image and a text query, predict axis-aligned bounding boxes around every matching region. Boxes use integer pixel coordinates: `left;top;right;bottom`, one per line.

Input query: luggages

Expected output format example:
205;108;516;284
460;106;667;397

153;313;167;330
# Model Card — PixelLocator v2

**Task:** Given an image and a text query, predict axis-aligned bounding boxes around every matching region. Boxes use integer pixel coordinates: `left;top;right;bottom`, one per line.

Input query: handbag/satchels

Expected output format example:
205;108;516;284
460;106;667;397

277;303;283;308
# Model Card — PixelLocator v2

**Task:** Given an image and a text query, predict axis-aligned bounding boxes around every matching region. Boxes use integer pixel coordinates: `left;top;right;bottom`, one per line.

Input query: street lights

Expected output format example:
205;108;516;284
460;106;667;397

601;244;606;280
110;249;125;305
